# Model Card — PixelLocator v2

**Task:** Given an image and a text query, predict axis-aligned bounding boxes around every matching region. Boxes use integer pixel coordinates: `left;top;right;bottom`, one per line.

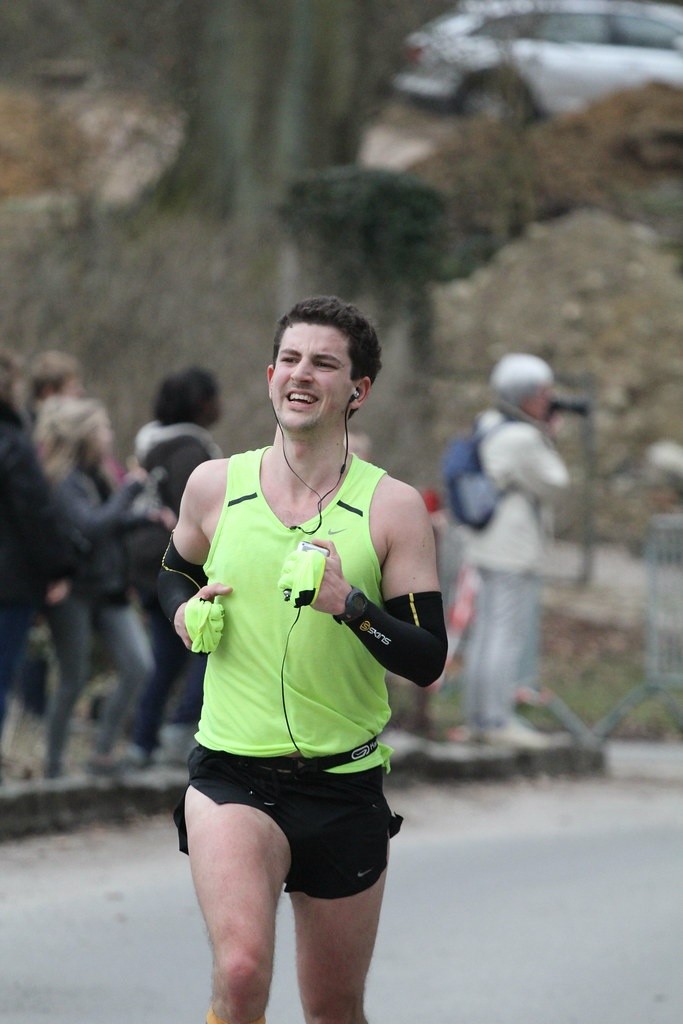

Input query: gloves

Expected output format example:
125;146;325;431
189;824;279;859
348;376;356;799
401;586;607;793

279;550;325;607
184;598;224;654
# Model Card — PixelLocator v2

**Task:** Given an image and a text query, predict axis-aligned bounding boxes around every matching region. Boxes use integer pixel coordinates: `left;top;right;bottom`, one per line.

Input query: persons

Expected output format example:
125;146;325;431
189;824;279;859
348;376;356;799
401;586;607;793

1;347;227;782
456;351;573;748
156;295;448;1024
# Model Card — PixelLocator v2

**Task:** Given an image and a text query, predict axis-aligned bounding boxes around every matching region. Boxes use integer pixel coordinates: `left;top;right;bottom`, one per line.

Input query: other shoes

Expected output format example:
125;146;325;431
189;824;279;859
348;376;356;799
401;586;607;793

472;720;545;751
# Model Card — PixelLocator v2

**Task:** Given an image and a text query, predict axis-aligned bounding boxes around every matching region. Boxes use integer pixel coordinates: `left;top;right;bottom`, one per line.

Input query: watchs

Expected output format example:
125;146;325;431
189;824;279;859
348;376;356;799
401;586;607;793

333;583;366;623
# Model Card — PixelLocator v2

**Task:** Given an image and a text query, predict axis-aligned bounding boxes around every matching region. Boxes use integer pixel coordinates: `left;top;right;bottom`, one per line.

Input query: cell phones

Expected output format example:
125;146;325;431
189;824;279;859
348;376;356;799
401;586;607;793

284;541;329;597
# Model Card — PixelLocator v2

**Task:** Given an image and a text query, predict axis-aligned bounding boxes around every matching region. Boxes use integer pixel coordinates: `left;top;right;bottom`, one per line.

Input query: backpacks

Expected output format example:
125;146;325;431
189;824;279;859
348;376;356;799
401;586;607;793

444;413;515;529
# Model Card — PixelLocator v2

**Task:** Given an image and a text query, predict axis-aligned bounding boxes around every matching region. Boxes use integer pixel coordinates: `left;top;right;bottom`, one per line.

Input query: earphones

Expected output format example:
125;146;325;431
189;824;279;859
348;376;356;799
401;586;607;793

347;388;361;404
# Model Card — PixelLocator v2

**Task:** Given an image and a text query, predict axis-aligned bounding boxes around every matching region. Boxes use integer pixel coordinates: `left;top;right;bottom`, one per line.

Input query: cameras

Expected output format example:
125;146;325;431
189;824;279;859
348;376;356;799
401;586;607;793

547;395;590;422
